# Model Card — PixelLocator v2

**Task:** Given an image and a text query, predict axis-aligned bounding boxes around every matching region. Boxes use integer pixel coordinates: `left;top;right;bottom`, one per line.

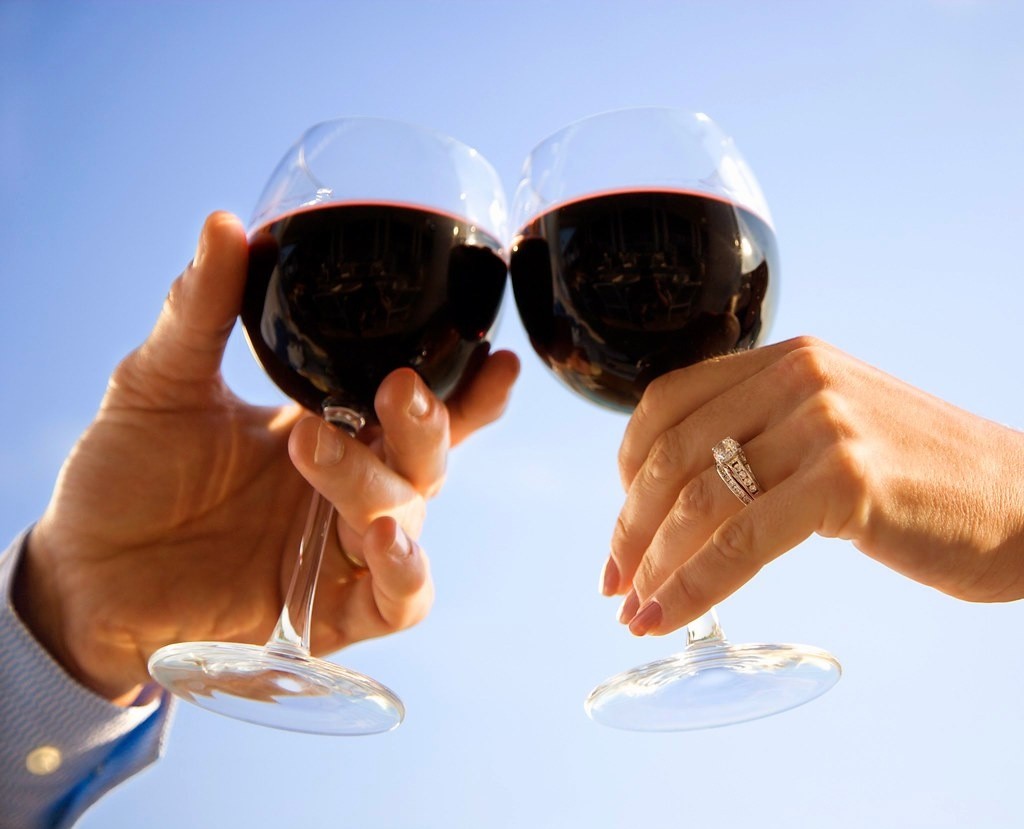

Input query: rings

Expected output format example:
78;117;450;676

711;435;765;509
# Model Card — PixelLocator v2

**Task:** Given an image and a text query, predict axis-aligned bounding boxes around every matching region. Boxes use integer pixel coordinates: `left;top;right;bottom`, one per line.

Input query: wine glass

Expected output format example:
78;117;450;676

505;109;845;737
144;114;511;738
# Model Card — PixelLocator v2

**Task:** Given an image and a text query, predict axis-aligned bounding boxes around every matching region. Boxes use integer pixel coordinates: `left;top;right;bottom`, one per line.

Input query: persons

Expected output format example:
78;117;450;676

1;206;519;828
598;334;1022;636
